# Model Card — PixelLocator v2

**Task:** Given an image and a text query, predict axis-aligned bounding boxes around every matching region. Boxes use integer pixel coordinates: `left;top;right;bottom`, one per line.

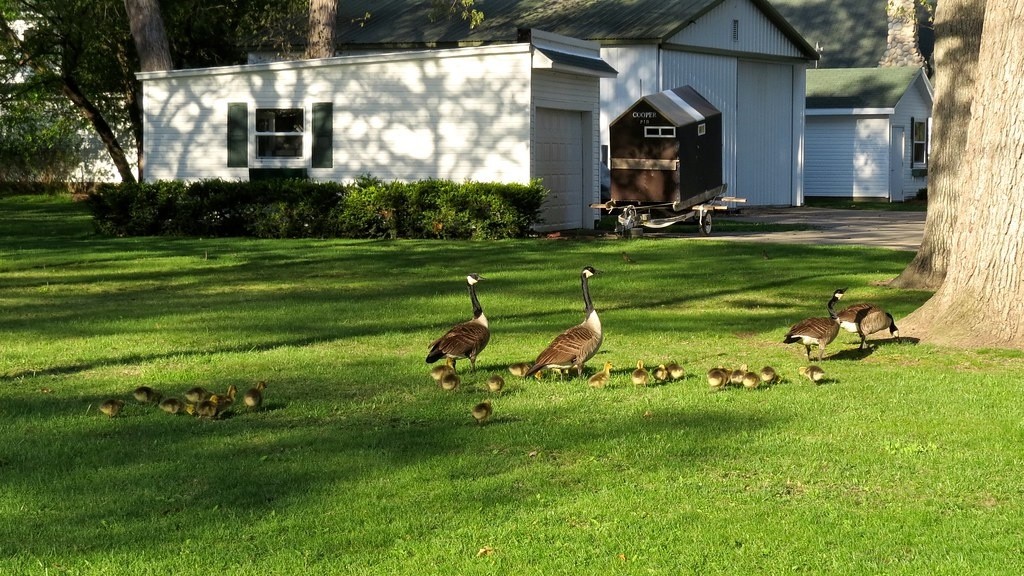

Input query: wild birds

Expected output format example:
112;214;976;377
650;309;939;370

631;360;684;387
429;357;460;390
488;376;504;393
133;387;161;405
472;400;492;424
98;399;125;418
783;286;849;361
425;273;491;371
588;361;616;388
243;381;267;409
159;384;239;419
798;366;824;381
622;251;636;263
523;266;604;379
508;363;543;380
706;363;776;390
837;302;901;351
